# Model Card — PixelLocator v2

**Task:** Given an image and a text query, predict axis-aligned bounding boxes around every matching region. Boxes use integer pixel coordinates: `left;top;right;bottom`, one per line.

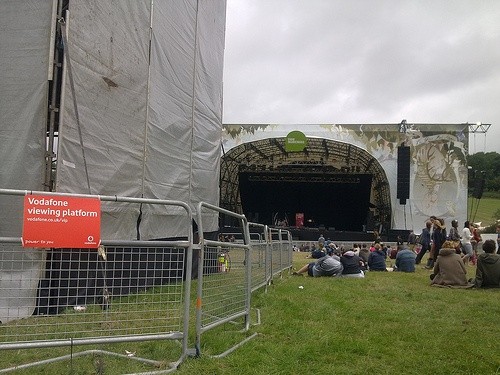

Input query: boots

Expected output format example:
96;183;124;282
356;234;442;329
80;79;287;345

422;259;434;270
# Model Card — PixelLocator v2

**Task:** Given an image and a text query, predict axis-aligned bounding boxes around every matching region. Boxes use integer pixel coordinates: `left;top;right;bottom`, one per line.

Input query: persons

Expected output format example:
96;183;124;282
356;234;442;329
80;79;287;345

292;215;500;290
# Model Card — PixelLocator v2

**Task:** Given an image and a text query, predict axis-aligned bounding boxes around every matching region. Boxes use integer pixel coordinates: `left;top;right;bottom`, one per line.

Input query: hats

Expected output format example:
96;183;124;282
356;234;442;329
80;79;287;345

320;234;323;236
374;243;380;248
362;243;367;246
442;240;454;248
380;244;383;246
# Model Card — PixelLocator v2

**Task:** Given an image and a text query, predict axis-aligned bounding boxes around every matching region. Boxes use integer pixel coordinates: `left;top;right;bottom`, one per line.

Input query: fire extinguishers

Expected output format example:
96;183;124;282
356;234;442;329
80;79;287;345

218;250;226;272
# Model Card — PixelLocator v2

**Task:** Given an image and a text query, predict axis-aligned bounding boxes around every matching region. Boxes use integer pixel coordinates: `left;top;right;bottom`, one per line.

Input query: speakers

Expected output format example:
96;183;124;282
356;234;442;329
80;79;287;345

396;145;411;204
473;171;486;198
